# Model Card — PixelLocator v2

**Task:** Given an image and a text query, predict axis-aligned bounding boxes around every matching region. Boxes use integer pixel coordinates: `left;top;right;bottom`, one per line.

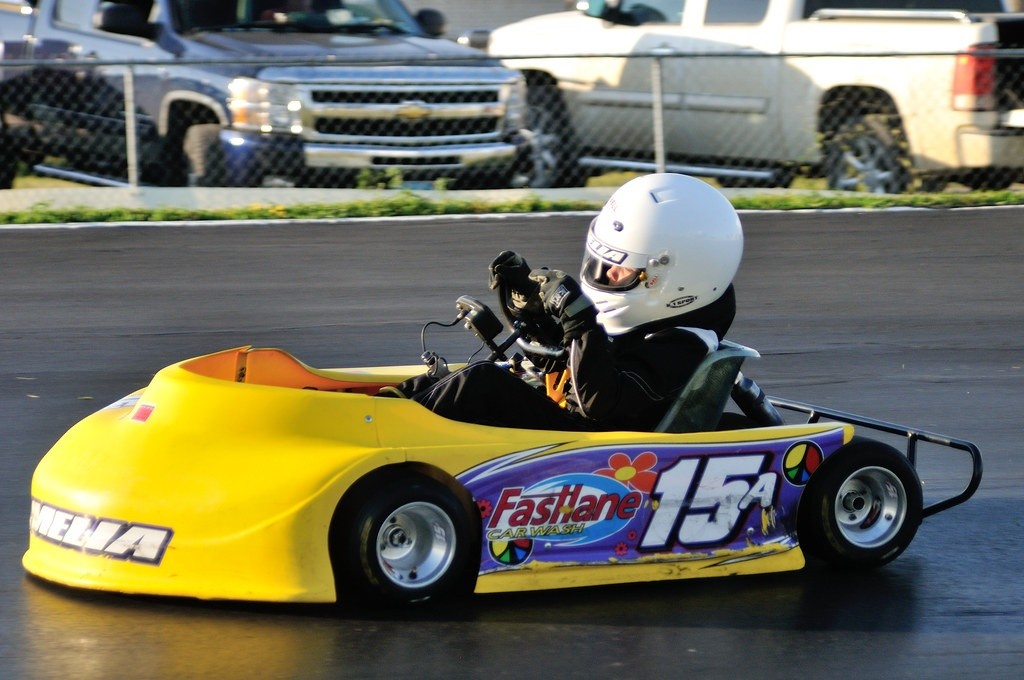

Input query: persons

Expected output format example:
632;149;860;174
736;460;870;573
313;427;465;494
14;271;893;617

369;173;744;432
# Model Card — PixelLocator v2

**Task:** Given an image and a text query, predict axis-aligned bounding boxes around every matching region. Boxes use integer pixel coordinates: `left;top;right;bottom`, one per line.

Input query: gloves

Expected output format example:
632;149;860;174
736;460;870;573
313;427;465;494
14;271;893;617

528;268;599;345
487;251;539;309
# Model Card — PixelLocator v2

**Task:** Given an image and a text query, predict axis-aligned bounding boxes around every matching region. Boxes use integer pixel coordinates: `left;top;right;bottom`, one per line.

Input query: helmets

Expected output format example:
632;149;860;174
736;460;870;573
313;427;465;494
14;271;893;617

581;172;744;335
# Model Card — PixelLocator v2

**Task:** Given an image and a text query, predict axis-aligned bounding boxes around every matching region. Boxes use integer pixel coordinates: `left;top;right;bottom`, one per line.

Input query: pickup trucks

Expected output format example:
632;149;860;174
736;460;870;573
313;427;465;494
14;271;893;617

486;0;1024;193
0;0;527;190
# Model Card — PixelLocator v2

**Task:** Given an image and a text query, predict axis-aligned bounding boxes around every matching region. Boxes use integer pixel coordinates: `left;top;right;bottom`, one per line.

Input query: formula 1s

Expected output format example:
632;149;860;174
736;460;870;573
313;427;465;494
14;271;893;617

22;271;984;621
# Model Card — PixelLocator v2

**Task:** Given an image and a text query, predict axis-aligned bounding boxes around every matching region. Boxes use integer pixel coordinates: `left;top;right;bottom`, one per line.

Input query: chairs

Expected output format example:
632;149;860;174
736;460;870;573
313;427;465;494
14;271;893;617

656;339;762;433
